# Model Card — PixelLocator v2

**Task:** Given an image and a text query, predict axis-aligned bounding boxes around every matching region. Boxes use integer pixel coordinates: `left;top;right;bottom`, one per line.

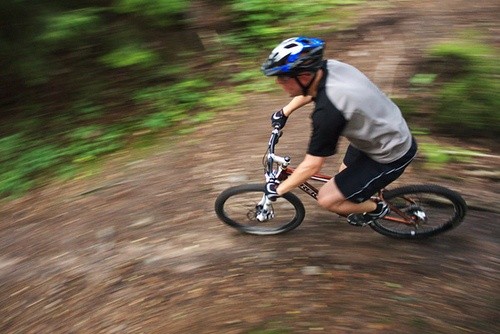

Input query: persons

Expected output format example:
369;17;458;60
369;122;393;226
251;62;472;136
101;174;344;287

260;37;418;227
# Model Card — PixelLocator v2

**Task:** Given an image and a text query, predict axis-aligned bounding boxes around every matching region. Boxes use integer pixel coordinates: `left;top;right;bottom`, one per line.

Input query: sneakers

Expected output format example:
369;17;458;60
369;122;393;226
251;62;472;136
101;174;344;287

347;197;391;227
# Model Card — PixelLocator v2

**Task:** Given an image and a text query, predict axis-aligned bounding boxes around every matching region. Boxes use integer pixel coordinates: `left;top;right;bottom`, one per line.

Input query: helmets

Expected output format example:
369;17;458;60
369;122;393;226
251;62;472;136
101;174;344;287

260;36;327;77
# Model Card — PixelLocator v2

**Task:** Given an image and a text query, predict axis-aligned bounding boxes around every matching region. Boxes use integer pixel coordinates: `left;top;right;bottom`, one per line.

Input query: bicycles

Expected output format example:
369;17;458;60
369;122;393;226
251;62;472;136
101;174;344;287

213;122;468;239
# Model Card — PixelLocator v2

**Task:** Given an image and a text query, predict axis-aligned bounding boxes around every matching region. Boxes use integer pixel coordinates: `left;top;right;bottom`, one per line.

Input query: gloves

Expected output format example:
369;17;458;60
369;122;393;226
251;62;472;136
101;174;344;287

271;108;288;130
265;183;279;202
